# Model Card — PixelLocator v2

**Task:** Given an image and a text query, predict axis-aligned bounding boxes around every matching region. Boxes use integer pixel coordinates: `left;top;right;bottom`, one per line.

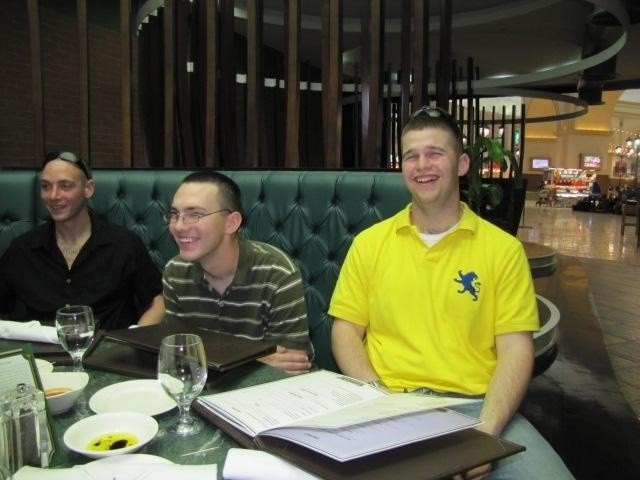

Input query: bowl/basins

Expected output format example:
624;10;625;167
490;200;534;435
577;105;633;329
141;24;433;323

64;410;159;459
37;371;90;417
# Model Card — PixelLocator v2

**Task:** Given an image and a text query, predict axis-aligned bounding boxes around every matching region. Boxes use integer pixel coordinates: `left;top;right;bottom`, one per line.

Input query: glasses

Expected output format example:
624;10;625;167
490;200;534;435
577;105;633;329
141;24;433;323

45;148;91;181
164;208;233;223
409;106;460;137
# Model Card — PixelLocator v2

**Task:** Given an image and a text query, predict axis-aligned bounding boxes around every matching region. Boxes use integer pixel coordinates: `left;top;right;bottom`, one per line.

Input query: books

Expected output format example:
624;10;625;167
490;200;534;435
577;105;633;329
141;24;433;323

193;369;530;480
80;322;278;380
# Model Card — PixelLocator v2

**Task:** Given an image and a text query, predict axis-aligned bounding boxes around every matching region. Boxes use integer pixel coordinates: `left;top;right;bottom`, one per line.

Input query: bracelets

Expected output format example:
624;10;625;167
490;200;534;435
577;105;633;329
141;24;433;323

368;379;386;388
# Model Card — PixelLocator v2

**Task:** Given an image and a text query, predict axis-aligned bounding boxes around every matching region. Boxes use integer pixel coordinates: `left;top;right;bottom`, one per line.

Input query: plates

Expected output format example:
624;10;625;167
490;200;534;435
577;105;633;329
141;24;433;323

87;380;185;416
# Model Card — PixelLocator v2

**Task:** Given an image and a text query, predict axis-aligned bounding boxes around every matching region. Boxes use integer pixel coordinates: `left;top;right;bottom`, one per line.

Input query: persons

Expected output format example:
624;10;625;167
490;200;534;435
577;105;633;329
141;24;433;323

335;105;576;480
0;142;166;334
163;170;312;377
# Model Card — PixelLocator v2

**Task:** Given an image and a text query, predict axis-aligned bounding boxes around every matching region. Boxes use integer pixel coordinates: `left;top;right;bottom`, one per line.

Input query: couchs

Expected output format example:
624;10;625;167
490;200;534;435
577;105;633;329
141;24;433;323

0;166;411;372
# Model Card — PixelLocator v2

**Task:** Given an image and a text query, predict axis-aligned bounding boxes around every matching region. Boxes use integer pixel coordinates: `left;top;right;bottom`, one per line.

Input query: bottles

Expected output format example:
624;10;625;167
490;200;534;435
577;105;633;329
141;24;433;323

12;382;42;473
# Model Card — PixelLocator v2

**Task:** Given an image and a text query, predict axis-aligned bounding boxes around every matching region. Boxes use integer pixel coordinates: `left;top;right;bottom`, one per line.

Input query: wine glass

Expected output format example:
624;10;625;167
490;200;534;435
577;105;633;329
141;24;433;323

158;335;207;437
54;306;94;374
